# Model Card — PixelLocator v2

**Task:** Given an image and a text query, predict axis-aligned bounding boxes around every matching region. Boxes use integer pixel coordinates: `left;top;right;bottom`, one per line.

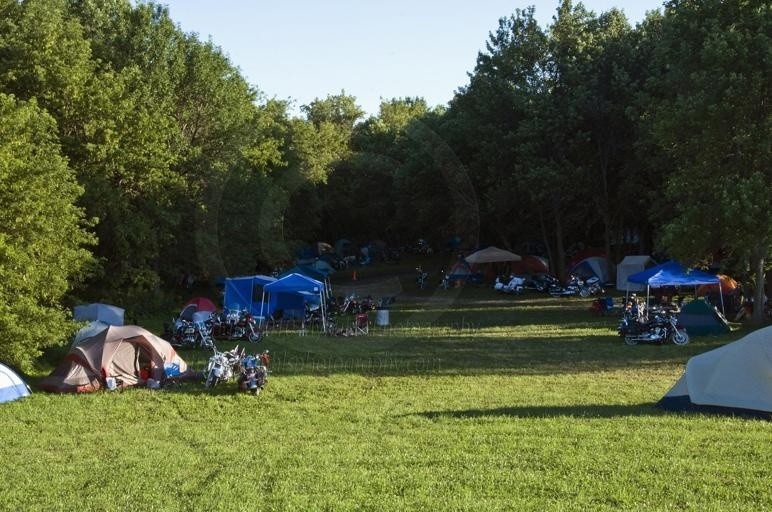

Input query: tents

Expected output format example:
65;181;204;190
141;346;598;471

567;249;600;275
570;256;616;287
445;257;483;285
312;260;337;274
1;361;33;404
517;253;551;275
676;299;731;335
697;274;738;296
41;322;188;392
183;295;217;312
52;303;124;361
654;322;771;421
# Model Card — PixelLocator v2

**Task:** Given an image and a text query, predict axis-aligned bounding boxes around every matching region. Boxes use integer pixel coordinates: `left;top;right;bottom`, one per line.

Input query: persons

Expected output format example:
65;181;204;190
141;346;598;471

655;287;664;304
666;287;673;302
734;302;752;321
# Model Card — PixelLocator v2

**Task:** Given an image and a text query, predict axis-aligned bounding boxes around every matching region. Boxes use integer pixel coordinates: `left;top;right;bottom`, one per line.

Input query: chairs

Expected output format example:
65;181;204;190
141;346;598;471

353;313;371;337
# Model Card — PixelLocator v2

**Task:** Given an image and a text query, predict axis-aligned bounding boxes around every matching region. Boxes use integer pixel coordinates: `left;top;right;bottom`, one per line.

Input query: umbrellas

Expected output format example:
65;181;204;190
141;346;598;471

464;244;521;265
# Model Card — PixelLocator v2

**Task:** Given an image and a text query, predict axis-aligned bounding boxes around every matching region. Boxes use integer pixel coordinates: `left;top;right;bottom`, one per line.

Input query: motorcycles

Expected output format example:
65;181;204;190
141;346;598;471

303;288;394;336
261;260;288;277
415;262;432;288
494;273;608;297
616;293;688;346
331;239;432;269
159;305;272;396
592;295;613;316
440;263;449;289
